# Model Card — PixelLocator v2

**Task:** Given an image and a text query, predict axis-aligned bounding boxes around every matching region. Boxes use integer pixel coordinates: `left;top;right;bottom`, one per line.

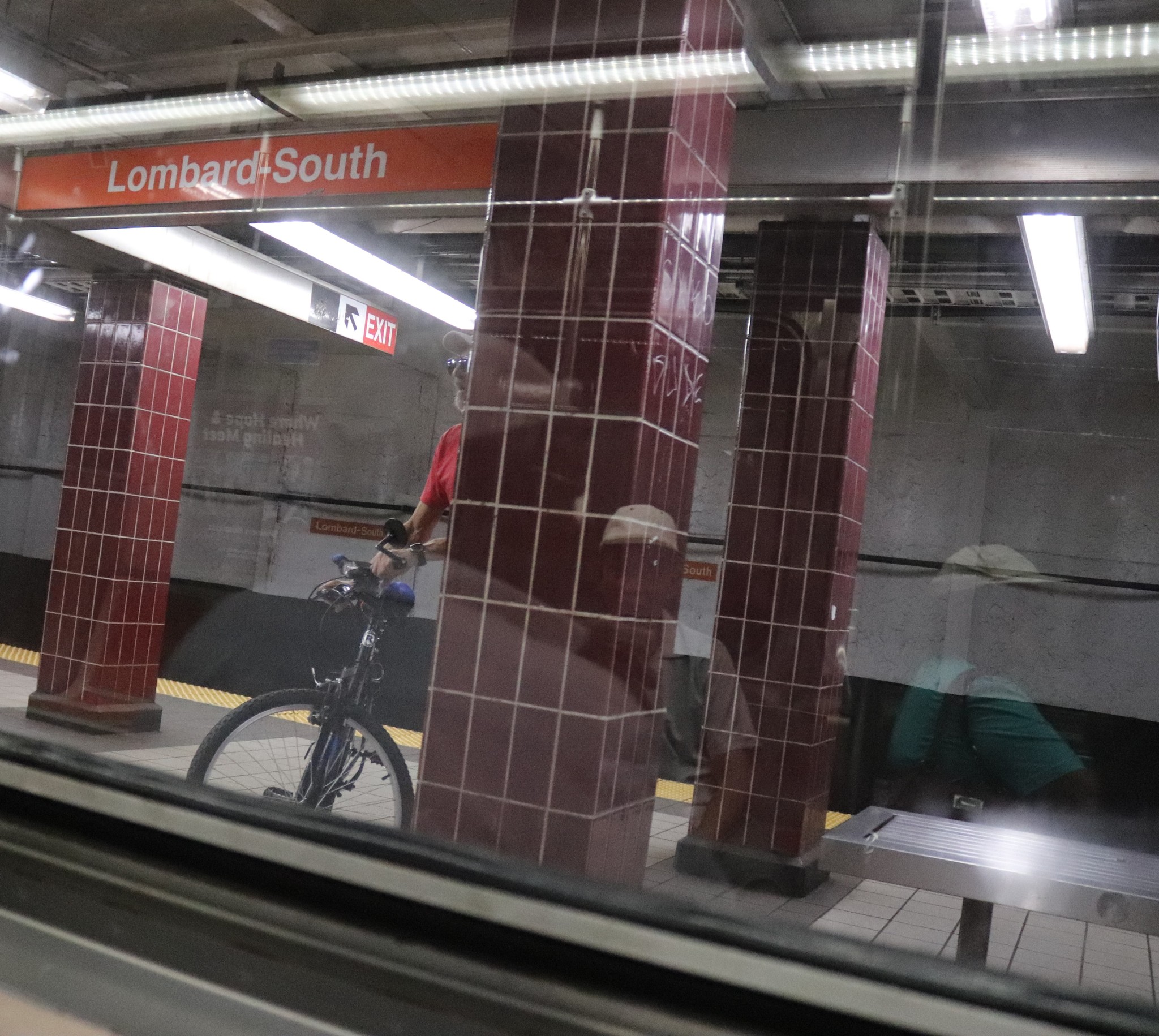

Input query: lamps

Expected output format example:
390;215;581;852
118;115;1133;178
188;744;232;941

978;0;1099;356
250;217;478;331
0;14;1159;149
0;270;80;325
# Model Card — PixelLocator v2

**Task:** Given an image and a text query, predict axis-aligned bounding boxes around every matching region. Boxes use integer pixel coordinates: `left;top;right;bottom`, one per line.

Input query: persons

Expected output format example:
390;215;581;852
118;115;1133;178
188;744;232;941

580;492;756;861
140;318;655;931
863;538;1098;892
365;349;471;585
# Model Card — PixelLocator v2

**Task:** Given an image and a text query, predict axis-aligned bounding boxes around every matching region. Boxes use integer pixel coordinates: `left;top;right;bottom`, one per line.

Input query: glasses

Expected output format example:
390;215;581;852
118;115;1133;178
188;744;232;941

445;356;472;375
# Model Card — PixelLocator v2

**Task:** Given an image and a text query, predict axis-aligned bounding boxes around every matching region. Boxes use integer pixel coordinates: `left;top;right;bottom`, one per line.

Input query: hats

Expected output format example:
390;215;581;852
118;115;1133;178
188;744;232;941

602;505;679;552
337;331;581;473
443;330;474;355
927;543;1055;597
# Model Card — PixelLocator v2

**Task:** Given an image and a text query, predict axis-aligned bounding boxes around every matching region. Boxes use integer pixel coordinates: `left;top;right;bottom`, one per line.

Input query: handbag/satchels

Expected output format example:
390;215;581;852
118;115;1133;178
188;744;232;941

874;666;1022;828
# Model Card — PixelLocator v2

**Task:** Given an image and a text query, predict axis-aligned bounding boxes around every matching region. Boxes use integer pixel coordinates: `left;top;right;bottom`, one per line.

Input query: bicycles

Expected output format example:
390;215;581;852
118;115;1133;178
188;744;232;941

184;512;419;833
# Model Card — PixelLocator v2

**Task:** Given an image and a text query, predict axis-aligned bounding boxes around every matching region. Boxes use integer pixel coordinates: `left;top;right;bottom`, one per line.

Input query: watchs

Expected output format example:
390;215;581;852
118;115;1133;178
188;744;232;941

410;542;430;569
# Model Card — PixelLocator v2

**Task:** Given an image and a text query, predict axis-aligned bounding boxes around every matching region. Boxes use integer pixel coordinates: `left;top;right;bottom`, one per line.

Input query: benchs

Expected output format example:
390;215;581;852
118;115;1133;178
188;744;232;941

818;806;1159;971
